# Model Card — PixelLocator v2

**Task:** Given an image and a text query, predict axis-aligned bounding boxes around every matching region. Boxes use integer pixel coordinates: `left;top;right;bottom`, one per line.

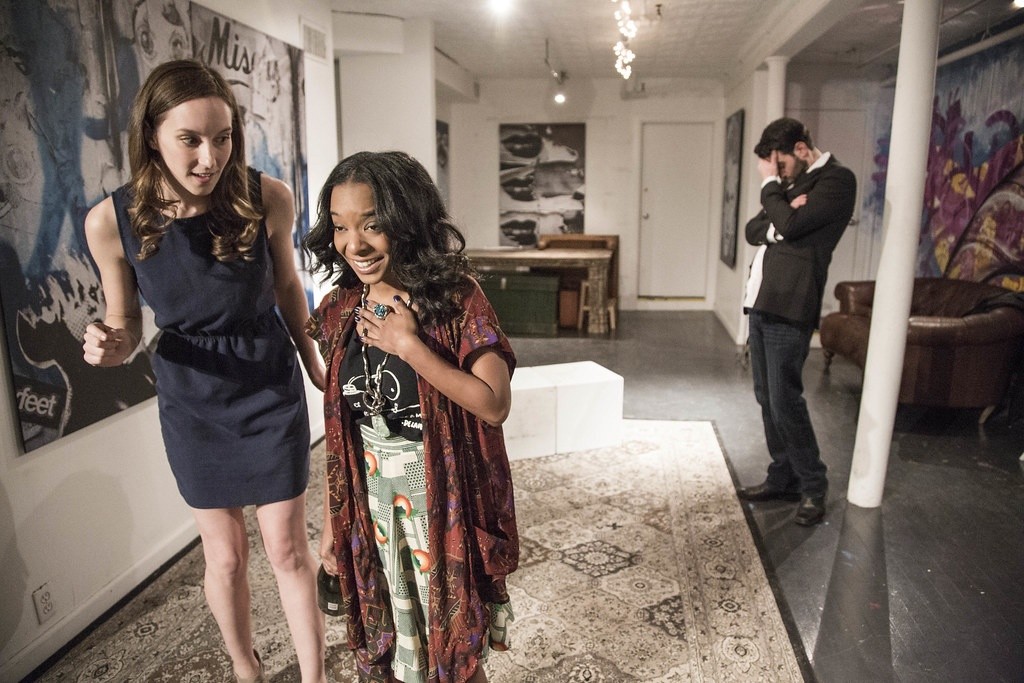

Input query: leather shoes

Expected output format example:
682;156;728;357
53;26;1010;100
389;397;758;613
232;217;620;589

795;489;825;526
736;475;802;503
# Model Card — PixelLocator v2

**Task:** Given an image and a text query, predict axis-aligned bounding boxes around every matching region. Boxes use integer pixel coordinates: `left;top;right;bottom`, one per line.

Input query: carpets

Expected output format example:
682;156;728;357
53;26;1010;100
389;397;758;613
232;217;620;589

31;418;818;683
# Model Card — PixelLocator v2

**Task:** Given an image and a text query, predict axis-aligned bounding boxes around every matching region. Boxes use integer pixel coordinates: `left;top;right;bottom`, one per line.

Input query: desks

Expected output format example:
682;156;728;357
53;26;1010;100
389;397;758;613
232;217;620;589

465;248;614;334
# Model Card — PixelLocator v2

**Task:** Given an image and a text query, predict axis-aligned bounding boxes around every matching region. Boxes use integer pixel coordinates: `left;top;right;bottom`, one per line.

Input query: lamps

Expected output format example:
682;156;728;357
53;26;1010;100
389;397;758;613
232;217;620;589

552;70;569;103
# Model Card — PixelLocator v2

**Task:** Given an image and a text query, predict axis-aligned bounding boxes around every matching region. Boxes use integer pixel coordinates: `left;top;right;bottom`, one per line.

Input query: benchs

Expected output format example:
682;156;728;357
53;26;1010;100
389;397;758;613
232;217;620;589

819;278;1024;421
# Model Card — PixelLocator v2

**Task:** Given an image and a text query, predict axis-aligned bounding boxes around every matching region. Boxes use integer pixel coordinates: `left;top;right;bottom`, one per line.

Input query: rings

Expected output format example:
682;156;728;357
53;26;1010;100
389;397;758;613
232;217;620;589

373;304;390;318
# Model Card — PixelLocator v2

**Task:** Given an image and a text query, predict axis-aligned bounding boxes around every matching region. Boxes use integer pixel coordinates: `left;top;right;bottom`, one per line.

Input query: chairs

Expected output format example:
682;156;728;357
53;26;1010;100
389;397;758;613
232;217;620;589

580;281;617;331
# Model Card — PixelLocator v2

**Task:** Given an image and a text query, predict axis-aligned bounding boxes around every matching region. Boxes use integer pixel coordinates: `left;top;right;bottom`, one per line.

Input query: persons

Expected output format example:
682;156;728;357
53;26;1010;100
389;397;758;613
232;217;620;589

83;59;333;682
299;151;519;682
737;117;857;525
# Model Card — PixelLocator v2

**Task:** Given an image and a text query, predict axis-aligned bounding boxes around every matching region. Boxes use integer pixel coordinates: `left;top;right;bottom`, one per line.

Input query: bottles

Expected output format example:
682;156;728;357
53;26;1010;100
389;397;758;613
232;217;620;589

316;565;345;615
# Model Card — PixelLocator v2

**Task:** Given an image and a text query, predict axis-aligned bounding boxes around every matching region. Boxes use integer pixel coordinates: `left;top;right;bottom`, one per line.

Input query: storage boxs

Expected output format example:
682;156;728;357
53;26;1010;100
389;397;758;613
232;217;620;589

500;367;558;463
560;290;579;327
534;360;624;452
473;269;565;335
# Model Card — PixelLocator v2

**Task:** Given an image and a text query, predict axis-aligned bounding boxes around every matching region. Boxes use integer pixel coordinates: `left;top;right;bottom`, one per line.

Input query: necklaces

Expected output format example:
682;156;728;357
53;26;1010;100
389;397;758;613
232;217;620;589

362;285;412;416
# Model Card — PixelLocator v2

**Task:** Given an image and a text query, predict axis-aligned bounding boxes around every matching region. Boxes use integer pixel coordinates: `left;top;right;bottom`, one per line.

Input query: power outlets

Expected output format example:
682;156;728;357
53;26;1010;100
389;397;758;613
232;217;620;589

33;581;59;625
